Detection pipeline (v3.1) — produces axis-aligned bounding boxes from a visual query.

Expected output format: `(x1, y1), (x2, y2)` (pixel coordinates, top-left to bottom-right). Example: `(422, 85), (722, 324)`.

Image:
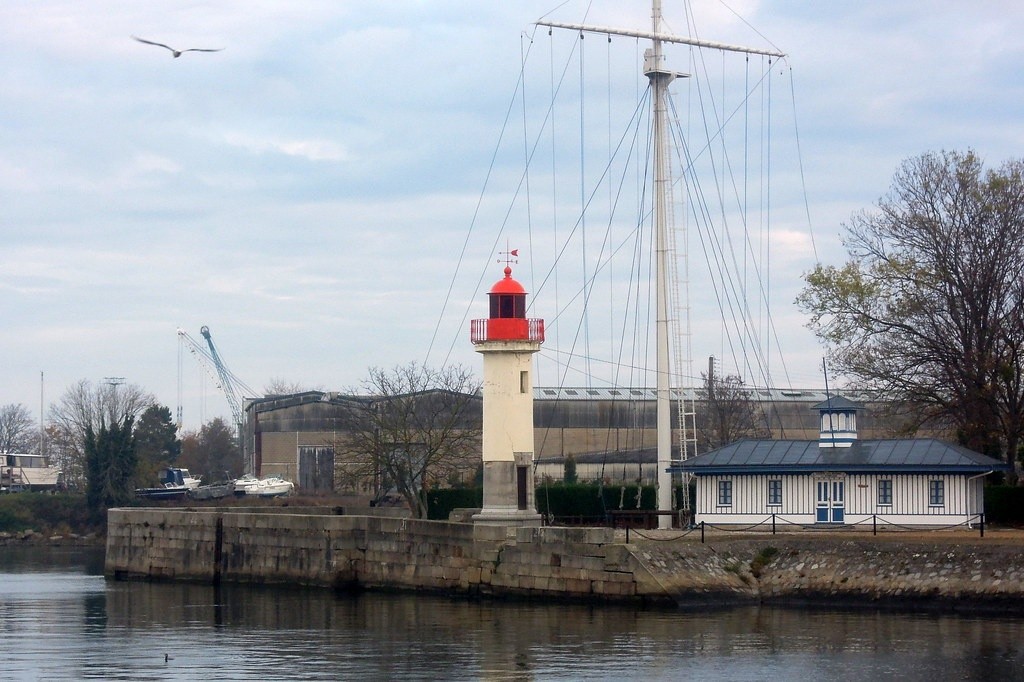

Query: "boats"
(0, 448), (296, 502)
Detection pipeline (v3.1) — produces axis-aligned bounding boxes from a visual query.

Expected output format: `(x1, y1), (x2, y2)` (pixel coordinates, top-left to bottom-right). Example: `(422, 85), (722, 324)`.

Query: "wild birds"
(164, 653), (175, 662)
(135, 36), (220, 58)
